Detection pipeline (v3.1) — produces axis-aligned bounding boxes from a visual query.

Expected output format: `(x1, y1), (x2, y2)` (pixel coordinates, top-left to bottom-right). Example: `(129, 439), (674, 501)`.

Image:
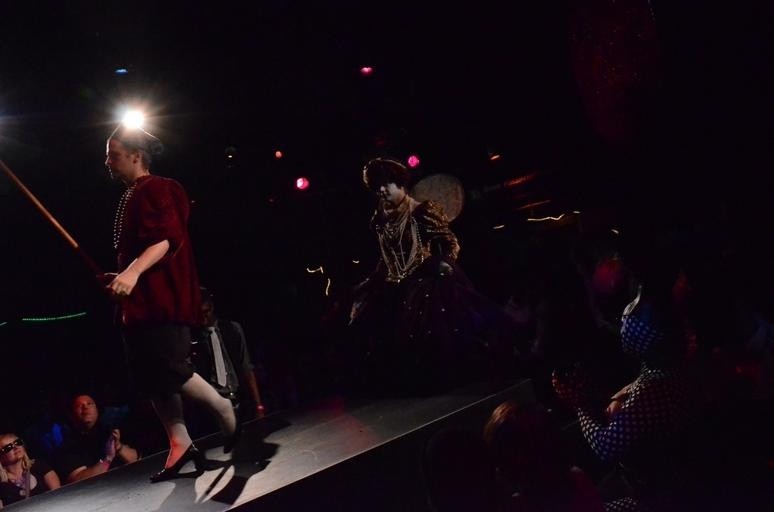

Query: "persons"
(346, 156), (462, 399)
(0, 256), (773, 512)
(95, 118), (239, 483)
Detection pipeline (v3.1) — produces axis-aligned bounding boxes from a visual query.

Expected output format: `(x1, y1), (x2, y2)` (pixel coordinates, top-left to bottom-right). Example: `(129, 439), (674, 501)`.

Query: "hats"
(360, 159), (410, 188)
(109, 123), (167, 159)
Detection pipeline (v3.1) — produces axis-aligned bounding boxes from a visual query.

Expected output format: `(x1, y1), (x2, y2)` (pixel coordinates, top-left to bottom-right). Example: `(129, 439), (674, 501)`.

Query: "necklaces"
(112, 184), (136, 250)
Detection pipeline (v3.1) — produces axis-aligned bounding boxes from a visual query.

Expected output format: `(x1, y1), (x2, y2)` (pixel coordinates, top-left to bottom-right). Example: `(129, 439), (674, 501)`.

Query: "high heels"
(224, 395), (243, 454)
(149, 442), (202, 482)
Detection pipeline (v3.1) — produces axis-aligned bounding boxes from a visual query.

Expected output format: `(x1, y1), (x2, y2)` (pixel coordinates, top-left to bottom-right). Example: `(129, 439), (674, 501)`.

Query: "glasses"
(0, 439), (24, 455)
